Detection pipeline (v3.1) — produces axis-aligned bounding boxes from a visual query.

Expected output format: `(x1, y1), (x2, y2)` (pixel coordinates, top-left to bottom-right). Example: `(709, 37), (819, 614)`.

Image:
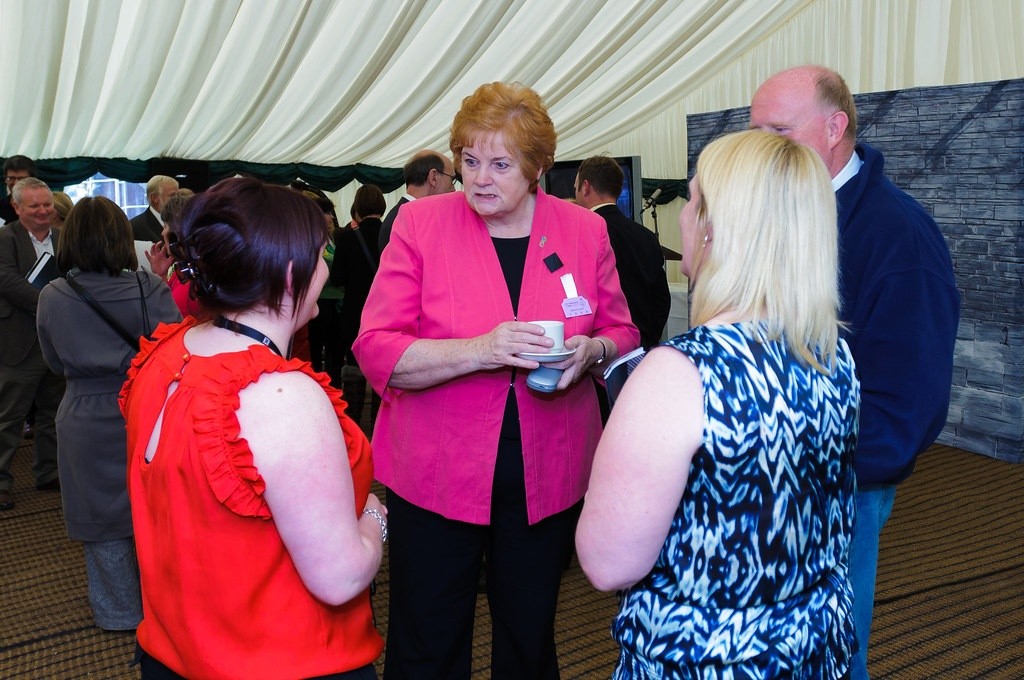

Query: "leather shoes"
(37, 476), (61, 491)
(0, 490), (16, 511)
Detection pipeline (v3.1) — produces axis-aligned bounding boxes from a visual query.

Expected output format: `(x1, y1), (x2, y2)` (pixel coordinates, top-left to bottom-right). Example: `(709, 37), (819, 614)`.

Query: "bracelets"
(363, 507), (388, 543)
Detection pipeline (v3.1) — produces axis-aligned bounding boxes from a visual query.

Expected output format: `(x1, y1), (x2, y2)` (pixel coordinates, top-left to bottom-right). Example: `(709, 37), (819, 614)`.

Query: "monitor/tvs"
(539, 156), (644, 226)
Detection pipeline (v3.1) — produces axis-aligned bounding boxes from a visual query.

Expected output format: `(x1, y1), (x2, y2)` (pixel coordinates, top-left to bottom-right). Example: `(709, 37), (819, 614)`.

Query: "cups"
(527, 321), (564, 354)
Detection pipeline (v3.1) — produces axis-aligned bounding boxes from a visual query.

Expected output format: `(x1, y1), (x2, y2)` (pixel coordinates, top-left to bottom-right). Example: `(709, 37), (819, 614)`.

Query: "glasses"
(436, 168), (458, 186)
(4, 176), (25, 184)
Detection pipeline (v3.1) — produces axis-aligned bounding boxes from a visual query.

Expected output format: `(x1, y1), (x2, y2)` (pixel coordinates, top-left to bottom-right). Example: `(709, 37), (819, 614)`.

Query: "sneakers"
(23, 421), (34, 440)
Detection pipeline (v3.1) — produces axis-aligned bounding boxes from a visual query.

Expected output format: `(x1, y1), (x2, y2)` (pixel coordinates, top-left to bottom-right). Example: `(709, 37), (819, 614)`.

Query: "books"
(25, 252), (58, 289)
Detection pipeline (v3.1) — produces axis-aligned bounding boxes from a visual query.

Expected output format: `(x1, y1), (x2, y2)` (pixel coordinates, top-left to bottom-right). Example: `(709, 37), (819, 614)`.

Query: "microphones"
(639, 189), (662, 215)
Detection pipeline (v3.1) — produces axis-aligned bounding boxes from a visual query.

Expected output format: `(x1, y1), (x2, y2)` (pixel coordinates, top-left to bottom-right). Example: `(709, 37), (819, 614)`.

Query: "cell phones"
(526, 366), (563, 392)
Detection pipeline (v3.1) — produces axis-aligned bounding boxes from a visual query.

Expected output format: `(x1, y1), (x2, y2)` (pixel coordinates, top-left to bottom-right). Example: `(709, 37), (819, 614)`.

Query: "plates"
(515, 346), (577, 363)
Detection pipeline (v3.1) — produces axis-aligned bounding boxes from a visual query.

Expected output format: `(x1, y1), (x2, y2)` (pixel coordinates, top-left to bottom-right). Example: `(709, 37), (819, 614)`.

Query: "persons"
(351, 82), (641, 680)
(378, 148), (457, 256)
(117, 177), (388, 680)
(0, 177), (73, 510)
(145, 188), (201, 317)
(0, 155), (36, 227)
(573, 156), (672, 354)
(751, 65), (962, 680)
(574, 128), (861, 680)
(36, 196), (183, 631)
(129, 175), (179, 250)
(290, 180), (381, 439)
